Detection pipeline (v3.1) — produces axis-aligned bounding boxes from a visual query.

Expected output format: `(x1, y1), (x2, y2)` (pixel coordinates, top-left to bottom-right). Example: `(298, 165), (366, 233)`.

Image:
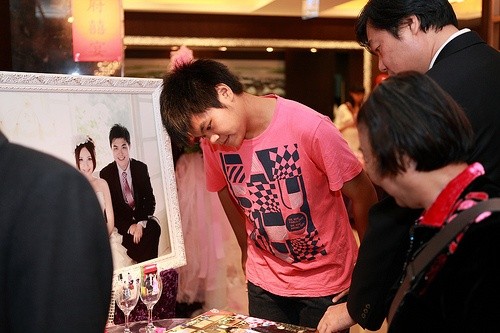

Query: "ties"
(122, 172), (134, 210)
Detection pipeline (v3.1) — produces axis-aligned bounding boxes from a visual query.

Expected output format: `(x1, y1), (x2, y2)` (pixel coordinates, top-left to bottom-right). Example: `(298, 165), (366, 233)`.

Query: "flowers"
(74, 134), (89, 148)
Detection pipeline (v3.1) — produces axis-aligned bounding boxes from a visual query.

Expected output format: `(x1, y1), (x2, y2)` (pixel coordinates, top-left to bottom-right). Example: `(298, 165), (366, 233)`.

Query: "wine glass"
(115, 277), (141, 333)
(139, 274), (164, 333)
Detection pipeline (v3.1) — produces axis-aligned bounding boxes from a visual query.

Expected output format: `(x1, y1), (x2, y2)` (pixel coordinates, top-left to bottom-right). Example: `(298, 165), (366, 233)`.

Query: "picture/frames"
(0, 71), (187, 278)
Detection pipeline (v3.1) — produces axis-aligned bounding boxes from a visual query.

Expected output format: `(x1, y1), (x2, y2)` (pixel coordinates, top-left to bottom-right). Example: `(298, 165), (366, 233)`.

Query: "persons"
(226, 325), (298, 333)
(74, 124), (161, 270)
(0, 132), (113, 333)
(316, 0), (500, 333)
(159, 57), (378, 333)
